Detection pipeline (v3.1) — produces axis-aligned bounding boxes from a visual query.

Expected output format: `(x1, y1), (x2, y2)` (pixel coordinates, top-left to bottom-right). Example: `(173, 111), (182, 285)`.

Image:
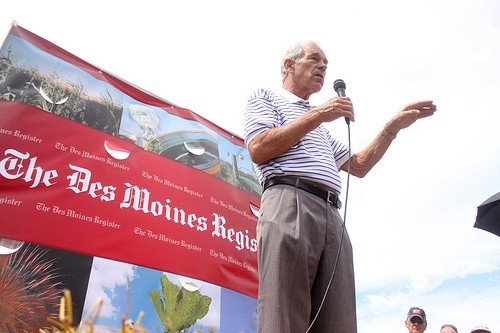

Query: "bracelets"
(382, 128), (397, 140)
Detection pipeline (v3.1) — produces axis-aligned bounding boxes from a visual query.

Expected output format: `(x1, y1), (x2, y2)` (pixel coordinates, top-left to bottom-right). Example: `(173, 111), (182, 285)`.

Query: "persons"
(440, 324), (458, 333)
(470, 325), (492, 333)
(243, 38), (438, 333)
(405, 306), (428, 333)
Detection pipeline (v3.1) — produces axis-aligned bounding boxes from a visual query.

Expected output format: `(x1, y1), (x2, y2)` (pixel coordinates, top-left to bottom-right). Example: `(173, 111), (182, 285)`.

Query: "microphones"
(333, 79), (350, 125)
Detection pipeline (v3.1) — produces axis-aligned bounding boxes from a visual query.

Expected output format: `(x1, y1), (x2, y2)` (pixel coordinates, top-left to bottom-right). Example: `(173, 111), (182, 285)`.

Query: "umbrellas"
(471, 192), (500, 237)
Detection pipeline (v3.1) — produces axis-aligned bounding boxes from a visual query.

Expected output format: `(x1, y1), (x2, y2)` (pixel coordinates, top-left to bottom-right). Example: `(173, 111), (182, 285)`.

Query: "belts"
(262, 178), (341, 208)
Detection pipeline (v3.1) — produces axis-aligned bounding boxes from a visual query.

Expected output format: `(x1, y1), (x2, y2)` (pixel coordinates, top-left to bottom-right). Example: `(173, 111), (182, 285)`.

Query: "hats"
(470, 326), (491, 333)
(407, 306), (426, 322)
